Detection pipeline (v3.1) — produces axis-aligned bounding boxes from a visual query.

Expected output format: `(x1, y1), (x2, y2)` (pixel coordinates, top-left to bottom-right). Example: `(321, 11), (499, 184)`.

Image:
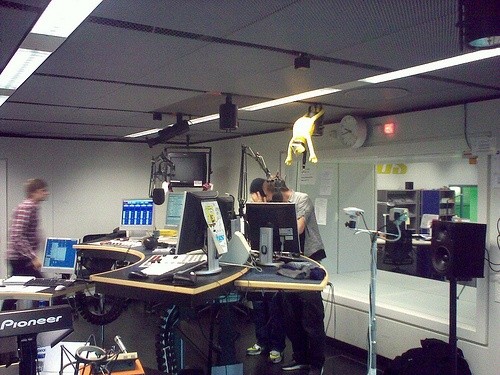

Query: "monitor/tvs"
(41, 238), (81, 279)
(245, 201), (301, 266)
(163, 191), (234, 275)
(120, 199), (154, 232)
(167, 151), (207, 186)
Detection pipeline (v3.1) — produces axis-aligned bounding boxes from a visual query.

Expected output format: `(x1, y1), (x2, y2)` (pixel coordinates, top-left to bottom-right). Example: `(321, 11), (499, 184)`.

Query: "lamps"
(455, 0), (500, 54)
(143, 118), (191, 148)
(219, 94), (239, 133)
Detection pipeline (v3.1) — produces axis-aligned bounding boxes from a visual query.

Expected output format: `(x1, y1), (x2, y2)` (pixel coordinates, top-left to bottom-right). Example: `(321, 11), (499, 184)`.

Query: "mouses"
(128, 271), (149, 280)
(54, 285), (66, 291)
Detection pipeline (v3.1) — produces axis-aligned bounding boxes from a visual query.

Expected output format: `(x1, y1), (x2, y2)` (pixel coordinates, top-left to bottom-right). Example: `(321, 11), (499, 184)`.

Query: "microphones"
(153, 173), (165, 205)
(272, 179), (283, 202)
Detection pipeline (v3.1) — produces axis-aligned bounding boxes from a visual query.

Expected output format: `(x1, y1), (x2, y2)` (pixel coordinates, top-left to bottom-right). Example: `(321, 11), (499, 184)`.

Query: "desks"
(377, 236), (431, 245)
(0, 232), (328, 375)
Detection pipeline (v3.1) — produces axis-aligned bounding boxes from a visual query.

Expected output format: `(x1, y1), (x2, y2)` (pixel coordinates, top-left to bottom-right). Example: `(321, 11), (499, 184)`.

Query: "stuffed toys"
(285, 108), (327, 167)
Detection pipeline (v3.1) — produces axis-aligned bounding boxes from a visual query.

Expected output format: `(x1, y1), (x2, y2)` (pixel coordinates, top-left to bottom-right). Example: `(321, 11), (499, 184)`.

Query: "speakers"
(430, 220), (487, 280)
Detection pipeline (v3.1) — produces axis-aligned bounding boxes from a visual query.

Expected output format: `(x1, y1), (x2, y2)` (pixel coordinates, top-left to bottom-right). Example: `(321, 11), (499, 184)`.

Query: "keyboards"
(153, 260), (207, 282)
(272, 254), (308, 264)
(23, 278), (76, 287)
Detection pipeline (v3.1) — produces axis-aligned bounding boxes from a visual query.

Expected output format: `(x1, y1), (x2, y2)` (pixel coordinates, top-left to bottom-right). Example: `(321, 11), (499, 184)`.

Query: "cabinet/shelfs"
(377, 189), (419, 233)
(418, 189), (456, 231)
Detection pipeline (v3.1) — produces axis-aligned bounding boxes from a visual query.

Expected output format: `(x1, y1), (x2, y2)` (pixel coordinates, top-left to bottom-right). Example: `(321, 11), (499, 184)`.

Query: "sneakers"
(268, 350), (284, 363)
(309, 365), (324, 375)
(247, 343), (269, 355)
(282, 357), (310, 370)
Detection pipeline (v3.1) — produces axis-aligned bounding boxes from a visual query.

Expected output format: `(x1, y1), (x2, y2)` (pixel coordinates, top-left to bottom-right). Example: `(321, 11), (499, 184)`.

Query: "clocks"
(340, 113), (367, 149)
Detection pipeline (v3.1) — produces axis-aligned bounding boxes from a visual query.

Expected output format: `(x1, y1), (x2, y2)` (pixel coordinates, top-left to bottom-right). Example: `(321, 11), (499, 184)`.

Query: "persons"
(1, 178), (49, 312)
(245, 178), (286, 362)
(262, 172), (327, 375)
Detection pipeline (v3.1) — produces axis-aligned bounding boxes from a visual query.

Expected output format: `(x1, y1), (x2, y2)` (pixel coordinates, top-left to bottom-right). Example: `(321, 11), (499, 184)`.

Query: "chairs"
(382, 231), (414, 274)
(75, 233), (122, 313)
